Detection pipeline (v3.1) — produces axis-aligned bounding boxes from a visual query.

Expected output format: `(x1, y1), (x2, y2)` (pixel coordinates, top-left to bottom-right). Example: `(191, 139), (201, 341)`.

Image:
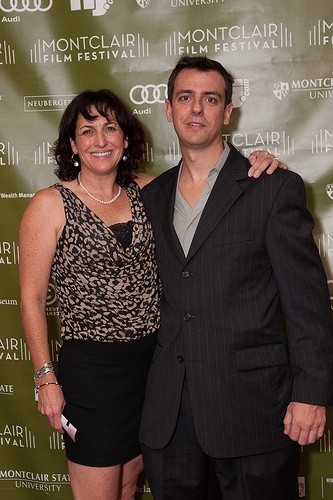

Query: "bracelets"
(36, 382), (62, 394)
(33, 360), (54, 381)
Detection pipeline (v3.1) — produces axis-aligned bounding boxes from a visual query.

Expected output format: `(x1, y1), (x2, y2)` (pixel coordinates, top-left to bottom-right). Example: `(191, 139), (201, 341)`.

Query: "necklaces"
(77, 170), (122, 205)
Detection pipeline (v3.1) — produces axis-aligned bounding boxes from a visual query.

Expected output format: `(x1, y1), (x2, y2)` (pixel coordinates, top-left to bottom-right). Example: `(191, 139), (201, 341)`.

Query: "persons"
(17, 88), (290, 500)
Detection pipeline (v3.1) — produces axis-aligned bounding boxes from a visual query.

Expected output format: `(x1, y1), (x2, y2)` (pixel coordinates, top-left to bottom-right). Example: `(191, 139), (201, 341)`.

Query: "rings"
(273, 157), (280, 162)
